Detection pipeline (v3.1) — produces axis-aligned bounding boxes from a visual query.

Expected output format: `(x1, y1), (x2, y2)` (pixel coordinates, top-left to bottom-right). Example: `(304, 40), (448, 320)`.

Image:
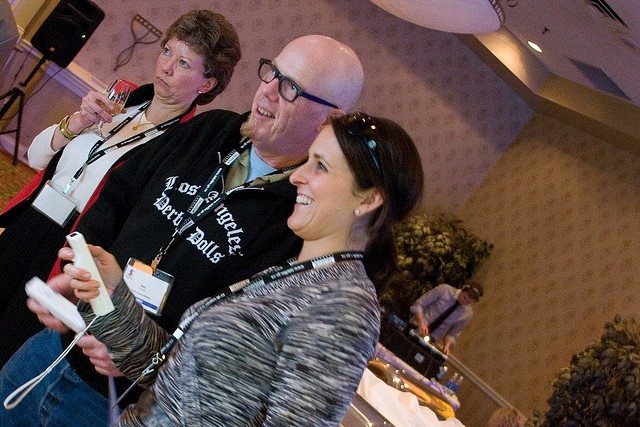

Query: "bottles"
(443, 372), (458, 388)
(454, 376), (464, 391)
(436, 366), (448, 379)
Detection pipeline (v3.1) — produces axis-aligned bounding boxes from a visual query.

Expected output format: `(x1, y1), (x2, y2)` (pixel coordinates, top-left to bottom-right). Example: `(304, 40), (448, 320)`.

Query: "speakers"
(31, 1), (106, 69)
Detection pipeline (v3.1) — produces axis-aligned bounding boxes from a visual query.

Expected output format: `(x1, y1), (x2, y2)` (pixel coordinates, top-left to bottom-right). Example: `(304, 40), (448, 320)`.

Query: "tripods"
(2, 54), (52, 169)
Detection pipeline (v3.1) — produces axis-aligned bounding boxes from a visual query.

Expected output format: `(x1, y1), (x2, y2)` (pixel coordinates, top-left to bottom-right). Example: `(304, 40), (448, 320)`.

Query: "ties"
(428, 299), (461, 334)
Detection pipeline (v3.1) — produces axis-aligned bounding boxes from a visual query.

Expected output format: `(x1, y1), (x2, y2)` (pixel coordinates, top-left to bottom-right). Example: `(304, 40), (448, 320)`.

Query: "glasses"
(257, 56), (340, 112)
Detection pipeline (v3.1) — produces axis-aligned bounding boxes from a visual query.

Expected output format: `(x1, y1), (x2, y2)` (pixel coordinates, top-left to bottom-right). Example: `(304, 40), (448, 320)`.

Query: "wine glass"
(88, 78), (132, 140)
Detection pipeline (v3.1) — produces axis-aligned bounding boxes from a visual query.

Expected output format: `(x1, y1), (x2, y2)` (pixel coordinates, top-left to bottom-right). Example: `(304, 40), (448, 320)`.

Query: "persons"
(410, 280), (484, 357)
(57, 112), (425, 427)
(0, 34), (364, 427)
(1, 10), (242, 365)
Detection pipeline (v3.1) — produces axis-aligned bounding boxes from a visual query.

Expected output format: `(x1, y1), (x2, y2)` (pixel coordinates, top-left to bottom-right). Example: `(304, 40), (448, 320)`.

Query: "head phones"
(462, 284), (481, 297)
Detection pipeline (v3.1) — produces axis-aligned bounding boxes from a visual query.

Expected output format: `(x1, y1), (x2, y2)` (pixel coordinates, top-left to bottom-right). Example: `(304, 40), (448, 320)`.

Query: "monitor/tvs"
(389, 315), (407, 332)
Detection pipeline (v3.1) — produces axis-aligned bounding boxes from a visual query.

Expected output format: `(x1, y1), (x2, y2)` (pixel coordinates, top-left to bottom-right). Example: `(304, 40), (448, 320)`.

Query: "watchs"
(59, 115), (77, 141)
